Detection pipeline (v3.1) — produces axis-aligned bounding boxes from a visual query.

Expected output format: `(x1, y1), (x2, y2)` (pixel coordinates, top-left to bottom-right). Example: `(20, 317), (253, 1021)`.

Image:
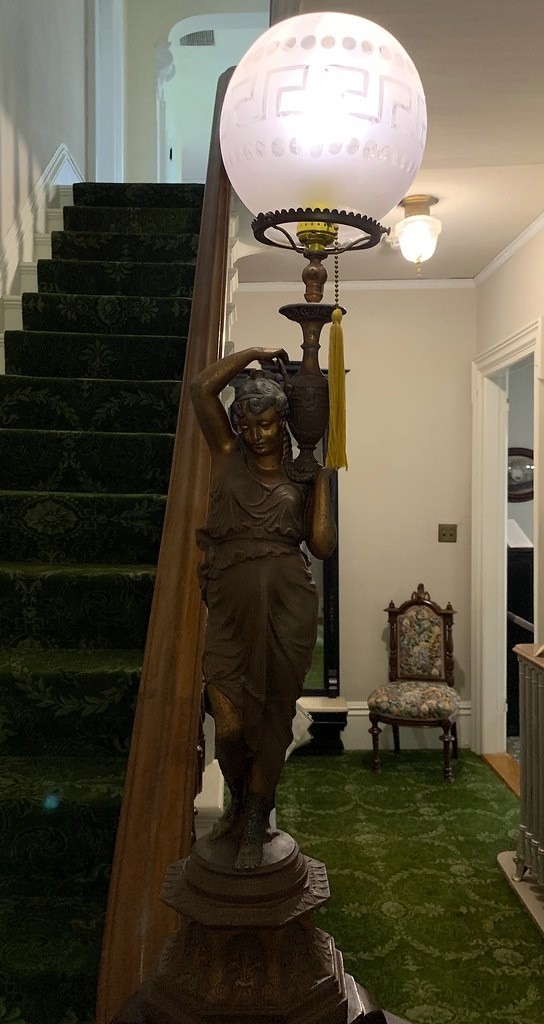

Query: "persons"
(189, 346), (337, 873)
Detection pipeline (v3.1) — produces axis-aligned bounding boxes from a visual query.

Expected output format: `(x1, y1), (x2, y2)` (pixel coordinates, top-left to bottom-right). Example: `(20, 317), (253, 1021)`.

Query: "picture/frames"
(506, 446), (534, 503)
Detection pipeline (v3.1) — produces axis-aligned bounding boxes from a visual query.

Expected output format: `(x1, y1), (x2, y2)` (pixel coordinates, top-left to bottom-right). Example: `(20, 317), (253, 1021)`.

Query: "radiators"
(513, 659), (544, 895)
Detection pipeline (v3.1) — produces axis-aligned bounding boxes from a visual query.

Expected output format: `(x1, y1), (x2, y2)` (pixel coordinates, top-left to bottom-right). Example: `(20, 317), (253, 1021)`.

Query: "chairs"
(368, 583), (458, 781)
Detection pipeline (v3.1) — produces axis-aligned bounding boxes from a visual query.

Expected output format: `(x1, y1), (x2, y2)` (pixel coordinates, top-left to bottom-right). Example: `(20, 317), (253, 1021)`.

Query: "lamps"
(220, 9), (429, 352)
(390, 195), (442, 277)
